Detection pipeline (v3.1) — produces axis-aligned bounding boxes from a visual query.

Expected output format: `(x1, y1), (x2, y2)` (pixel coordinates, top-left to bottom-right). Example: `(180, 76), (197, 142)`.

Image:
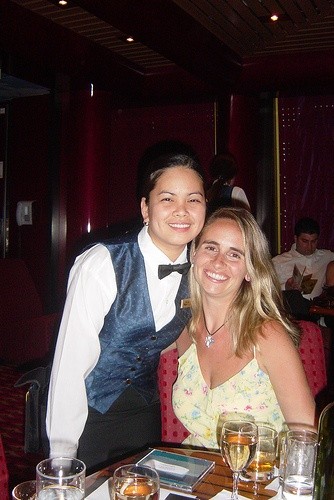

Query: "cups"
(111, 464), (161, 500)
(237, 424), (279, 481)
(274, 429), (318, 500)
(11, 456), (86, 500)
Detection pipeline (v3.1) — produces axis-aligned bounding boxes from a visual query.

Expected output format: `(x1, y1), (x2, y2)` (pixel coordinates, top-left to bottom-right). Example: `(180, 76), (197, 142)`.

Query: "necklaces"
(202, 303), (233, 349)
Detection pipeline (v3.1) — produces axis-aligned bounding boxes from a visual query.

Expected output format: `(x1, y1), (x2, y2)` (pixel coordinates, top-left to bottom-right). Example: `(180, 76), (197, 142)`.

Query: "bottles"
(313, 401), (334, 500)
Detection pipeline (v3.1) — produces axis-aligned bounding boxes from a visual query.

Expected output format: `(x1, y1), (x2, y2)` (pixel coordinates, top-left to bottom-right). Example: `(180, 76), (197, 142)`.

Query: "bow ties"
(158, 262), (192, 280)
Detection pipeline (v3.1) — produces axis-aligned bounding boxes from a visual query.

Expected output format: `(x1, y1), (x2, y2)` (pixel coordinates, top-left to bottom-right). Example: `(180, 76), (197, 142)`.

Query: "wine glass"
(220, 421), (258, 500)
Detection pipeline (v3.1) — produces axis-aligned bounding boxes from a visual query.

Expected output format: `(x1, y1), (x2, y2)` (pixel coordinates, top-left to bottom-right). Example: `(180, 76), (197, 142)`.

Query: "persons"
(171, 207), (319, 453)
(206, 152), (252, 213)
(44, 153), (207, 477)
(272, 217), (334, 327)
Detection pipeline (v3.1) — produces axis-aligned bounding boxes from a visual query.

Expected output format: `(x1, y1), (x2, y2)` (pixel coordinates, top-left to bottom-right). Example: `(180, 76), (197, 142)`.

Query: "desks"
(84, 446), (279, 500)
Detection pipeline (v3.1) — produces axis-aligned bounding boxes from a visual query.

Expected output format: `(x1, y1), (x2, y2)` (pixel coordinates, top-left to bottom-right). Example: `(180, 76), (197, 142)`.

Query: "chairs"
(0, 259), (330, 488)
